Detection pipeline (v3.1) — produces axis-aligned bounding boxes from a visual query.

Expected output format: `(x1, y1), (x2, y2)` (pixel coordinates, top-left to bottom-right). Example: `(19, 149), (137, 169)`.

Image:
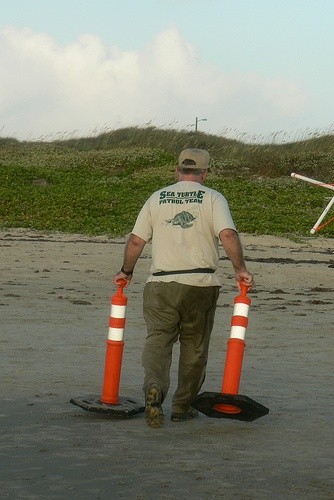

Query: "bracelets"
(121, 265), (133, 275)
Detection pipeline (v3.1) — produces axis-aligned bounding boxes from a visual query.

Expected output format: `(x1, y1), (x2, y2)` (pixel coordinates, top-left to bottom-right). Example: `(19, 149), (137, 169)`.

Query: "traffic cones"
(187, 281), (269, 422)
(69, 279), (146, 415)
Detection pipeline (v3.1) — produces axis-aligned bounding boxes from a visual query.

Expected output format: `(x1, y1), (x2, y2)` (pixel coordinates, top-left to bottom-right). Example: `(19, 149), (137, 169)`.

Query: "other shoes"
(170, 405), (200, 423)
(144, 385), (164, 428)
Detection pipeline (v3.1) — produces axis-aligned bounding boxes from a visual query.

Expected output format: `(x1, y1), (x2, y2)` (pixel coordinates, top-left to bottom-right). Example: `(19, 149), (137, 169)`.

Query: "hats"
(179, 148), (210, 170)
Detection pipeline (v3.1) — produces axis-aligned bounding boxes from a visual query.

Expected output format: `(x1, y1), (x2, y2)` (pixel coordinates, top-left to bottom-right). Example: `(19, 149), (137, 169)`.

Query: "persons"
(113, 148), (256, 428)
